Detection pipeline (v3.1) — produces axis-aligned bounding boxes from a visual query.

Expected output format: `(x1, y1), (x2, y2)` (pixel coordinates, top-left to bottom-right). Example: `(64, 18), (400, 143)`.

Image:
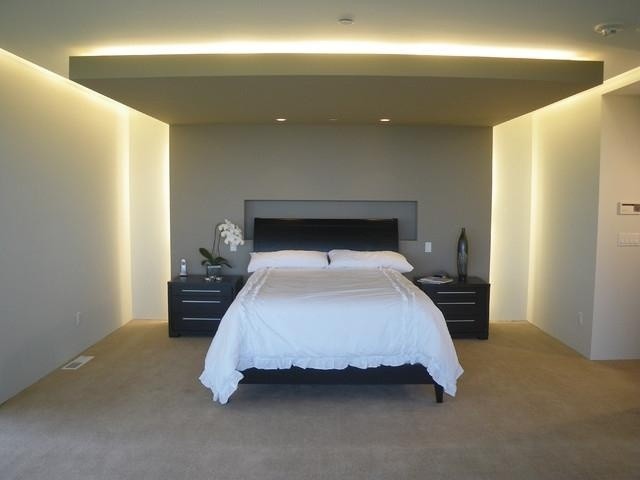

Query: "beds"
(198, 217), (467, 404)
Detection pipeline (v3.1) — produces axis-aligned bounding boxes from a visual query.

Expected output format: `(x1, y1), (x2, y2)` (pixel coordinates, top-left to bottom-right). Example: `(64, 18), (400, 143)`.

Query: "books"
(417, 276), (453, 285)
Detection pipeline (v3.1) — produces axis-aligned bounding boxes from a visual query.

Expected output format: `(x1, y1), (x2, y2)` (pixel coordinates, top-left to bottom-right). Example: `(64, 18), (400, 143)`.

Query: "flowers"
(197, 216), (245, 269)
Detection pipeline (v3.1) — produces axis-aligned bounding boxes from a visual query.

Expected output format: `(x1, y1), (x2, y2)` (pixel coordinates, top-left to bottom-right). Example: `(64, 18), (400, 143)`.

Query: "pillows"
(248, 248), (329, 273)
(326, 248), (414, 274)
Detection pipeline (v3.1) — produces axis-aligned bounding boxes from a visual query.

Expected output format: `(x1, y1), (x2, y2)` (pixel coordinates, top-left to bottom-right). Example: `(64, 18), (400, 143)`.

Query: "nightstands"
(413, 277), (490, 339)
(168, 272), (245, 338)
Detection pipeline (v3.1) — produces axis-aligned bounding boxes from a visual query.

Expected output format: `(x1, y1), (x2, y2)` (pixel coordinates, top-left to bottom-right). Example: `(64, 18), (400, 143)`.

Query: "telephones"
(179, 259), (188, 277)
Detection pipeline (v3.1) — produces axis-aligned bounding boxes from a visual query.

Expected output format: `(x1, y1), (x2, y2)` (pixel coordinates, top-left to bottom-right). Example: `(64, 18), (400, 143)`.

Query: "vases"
(457, 225), (469, 281)
(207, 264), (221, 278)
